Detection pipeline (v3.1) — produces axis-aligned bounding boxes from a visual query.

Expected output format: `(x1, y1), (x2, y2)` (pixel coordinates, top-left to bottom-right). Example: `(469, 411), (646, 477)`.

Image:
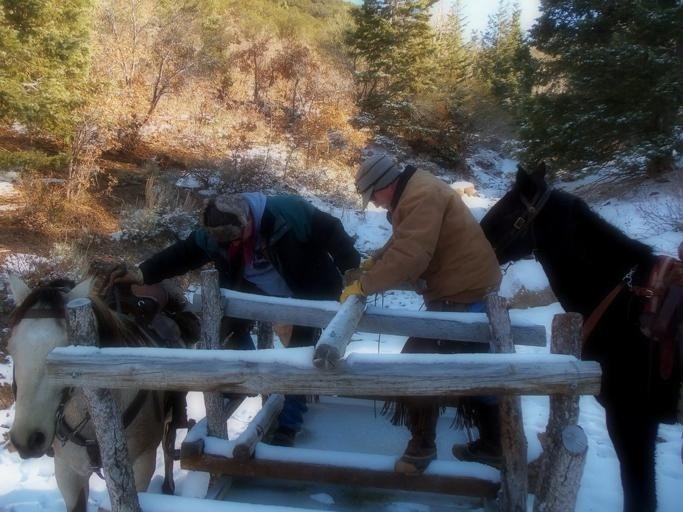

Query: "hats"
(204, 193), (249, 244)
(355, 152), (401, 210)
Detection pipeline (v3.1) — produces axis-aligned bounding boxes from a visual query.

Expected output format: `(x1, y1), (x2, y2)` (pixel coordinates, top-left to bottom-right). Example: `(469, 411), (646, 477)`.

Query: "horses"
(478, 160), (683, 512)
(5, 268), (193, 512)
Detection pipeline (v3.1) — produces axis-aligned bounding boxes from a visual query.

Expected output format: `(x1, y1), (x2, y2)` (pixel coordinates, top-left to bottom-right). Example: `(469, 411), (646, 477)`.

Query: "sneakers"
(394, 438), (436, 479)
(271, 423), (296, 448)
(452, 444), (505, 470)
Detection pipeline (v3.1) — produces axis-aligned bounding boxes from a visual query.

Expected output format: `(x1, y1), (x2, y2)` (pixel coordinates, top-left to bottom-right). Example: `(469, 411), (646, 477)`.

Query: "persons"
(340, 153), (504, 476)
(110, 192), (360, 444)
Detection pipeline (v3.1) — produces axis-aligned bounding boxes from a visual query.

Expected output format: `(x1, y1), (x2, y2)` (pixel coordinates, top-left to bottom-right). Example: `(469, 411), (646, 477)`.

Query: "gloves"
(339, 279), (367, 304)
